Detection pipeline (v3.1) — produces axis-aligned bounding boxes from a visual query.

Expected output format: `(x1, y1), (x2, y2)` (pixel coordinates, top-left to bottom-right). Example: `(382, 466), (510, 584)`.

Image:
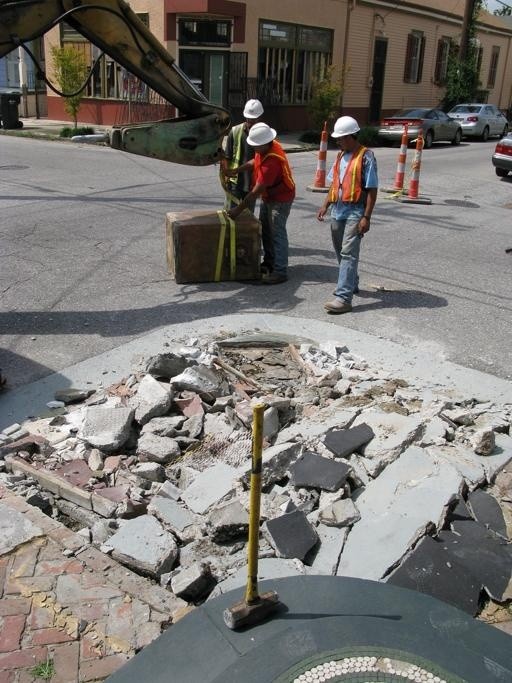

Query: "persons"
(316, 115), (378, 314)
(218, 99), (265, 213)
(225, 121), (296, 284)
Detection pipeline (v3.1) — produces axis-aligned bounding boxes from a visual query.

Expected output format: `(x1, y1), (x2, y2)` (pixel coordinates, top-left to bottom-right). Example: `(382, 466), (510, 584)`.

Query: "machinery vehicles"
(2, 0), (235, 167)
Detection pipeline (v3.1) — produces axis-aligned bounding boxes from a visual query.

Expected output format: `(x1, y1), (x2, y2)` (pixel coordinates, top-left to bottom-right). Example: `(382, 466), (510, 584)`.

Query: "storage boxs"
(163, 208), (262, 286)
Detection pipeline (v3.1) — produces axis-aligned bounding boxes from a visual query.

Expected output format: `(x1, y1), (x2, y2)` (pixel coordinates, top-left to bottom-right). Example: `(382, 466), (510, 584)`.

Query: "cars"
(446, 101), (509, 141)
(491, 131), (512, 177)
(376, 105), (461, 148)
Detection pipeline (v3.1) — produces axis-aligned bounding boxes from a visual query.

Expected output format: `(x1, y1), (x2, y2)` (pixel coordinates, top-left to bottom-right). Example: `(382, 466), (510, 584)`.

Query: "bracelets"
(362, 215), (371, 221)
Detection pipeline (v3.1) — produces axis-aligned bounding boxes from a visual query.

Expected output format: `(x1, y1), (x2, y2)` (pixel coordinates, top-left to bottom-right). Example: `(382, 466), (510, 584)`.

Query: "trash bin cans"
(507, 107), (512, 132)
(0, 92), (23, 130)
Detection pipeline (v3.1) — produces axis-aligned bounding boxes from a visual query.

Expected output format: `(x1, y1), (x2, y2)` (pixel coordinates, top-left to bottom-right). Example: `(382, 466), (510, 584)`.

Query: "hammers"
(222, 402), (280, 630)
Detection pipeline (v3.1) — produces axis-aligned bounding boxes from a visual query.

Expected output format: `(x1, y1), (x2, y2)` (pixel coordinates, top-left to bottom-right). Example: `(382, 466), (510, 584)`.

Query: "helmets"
(330, 115), (361, 138)
(243, 98), (265, 120)
(246, 122), (277, 146)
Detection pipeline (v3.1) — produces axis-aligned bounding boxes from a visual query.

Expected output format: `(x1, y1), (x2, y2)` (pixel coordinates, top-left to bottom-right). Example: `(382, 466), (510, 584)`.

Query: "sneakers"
(261, 262), (287, 284)
(324, 299), (352, 313)
(333, 284), (359, 296)
